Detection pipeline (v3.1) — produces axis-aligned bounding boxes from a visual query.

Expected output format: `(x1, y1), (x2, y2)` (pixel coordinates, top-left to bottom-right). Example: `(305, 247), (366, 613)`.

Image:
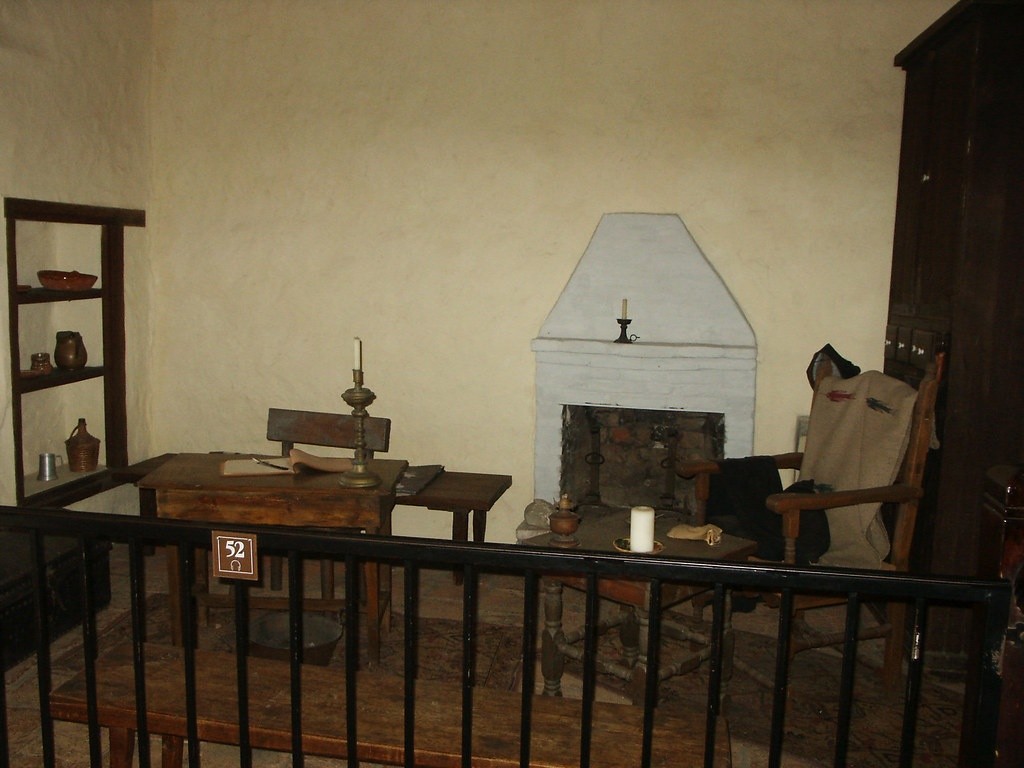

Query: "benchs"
(46, 641), (771, 768)
(111, 453), (513, 592)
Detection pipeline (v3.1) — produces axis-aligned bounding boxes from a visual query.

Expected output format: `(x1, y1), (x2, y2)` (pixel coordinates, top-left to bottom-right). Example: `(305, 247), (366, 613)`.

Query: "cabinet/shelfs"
(862, 0), (1024, 672)
(3, 196), (146, 510)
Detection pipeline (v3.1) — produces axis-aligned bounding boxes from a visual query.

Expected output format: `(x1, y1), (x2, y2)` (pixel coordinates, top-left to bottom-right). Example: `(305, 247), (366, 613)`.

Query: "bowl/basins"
(37, 270), (98, 291)
(249, 613), (344, 667)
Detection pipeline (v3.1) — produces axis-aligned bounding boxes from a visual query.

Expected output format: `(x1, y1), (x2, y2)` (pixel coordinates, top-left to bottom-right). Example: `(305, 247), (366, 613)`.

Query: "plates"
(613, 537), (664, 555)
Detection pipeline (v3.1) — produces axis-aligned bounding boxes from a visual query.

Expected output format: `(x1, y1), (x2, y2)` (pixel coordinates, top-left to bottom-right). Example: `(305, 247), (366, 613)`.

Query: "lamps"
(622, 297), (627, 320)
(354, 336), (361, 370)
(630, 505), (655, 553)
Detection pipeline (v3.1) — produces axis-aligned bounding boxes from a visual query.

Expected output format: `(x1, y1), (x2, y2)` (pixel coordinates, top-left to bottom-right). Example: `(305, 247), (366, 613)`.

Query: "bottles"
(65, 418), (101, 472)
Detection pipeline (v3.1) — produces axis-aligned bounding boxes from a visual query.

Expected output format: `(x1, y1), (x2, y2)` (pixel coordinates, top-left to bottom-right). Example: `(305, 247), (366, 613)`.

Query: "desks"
(135, 452), (409, 668)
(519, 509), (759, 715)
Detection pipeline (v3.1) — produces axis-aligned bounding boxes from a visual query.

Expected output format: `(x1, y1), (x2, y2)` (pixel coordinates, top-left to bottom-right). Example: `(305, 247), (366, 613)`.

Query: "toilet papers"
(629, 506), (654, 552)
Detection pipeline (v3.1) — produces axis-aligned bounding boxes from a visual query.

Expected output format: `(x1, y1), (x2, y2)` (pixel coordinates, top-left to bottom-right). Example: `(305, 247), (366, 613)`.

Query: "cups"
(37, 453), (63, 482)
(54, 331), (87, 371)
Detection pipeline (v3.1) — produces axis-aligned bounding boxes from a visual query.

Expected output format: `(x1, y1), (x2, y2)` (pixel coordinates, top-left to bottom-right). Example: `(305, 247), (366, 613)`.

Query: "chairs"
(267, 407), (393, 668)
(673, 352), (945, 733)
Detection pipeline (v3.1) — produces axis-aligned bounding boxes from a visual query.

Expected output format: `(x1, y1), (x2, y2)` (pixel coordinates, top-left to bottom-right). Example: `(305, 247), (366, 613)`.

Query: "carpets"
(532, 607), (962, 768)
(50, 589), (524, 697)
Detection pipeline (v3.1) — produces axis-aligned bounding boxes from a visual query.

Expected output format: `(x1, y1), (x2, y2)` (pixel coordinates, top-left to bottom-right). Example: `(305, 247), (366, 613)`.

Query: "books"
(220, 449), (354, 476)
